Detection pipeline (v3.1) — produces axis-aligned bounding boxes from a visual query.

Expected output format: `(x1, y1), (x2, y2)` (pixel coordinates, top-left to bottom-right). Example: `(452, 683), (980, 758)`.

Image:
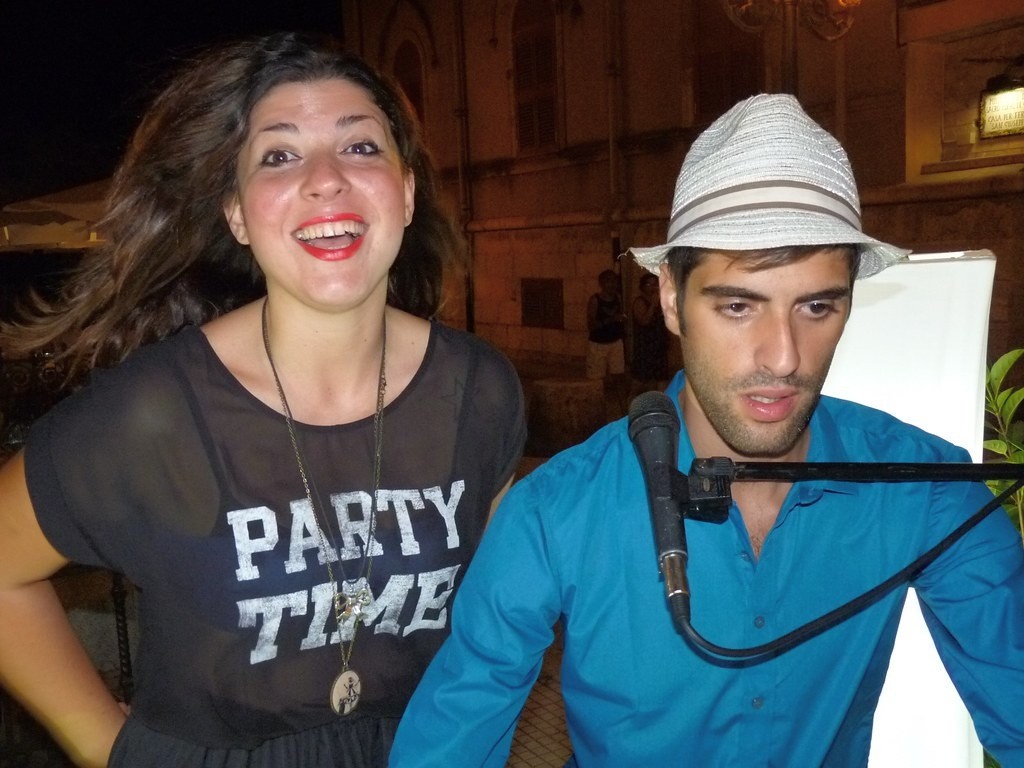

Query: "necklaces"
(262, 297), (389, 720)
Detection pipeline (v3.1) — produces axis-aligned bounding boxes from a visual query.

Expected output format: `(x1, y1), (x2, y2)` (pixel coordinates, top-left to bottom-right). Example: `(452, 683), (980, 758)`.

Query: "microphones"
(627, 392), (692, 623)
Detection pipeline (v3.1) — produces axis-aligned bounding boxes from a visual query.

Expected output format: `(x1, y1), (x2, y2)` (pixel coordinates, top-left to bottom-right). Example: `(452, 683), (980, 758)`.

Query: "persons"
(387, 95), (1024, 768)
(0, 34), (529, 768)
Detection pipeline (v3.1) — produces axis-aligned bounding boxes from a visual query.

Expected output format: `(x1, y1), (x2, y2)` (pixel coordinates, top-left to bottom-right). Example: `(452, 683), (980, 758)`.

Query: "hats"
(627, 93), (913, 283)
